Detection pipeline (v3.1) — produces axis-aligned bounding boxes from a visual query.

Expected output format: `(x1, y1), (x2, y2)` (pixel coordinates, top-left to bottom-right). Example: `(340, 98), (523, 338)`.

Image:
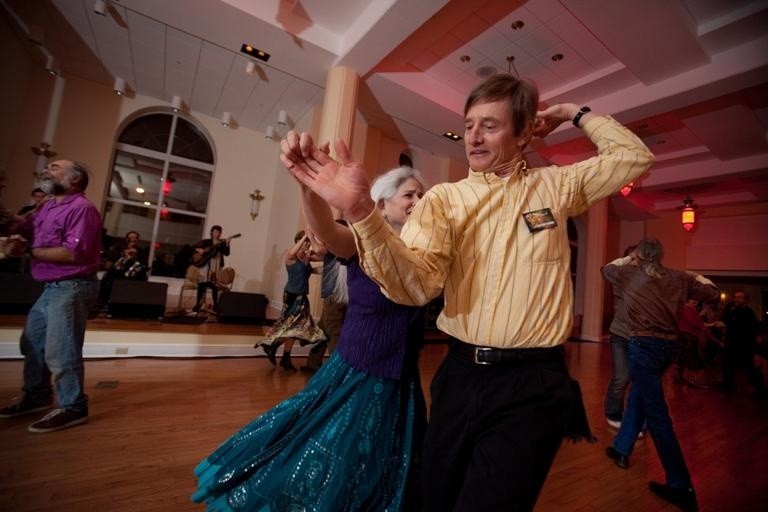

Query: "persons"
(189, 224), (232, 313)
(188, 129), (443, 511)
(597, 233), (768, 512)
(0, 188), (150, 321)
(300, 218), (352, 376)
(0, 158), (104, 433)
(278, 71), (658, 510)
(251, 225), (328, 375)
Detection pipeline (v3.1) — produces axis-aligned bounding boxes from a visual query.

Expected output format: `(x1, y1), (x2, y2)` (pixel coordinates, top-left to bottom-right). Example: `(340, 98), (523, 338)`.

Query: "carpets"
(0, 328), (331, 360)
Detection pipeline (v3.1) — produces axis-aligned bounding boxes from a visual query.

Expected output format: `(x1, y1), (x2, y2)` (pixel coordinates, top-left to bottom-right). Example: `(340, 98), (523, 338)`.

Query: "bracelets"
(571, 105), (592, 129)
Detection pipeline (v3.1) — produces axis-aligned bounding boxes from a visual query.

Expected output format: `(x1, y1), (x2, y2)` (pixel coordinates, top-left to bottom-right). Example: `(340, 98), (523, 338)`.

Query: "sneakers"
(27, 404), (88, 433)
(0, 389), (53, 418)
(300, 367), (316, 373)
(638, 431), (645, 439)
(605, 413), (623, 428)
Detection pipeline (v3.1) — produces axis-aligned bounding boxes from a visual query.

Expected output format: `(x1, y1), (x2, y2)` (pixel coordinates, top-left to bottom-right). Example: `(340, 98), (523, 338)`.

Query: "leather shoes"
(606, 446), (627, 468)
(649, 480), (697, 511)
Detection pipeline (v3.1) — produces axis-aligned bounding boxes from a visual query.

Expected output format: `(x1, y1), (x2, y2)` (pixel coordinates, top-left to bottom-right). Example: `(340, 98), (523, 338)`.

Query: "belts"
(448, 336), (558, 366)
(629, 330), (678, 341)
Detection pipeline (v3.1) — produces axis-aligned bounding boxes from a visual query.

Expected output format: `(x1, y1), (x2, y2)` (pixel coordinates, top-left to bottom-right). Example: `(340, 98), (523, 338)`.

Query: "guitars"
(192, 233), (242, 268)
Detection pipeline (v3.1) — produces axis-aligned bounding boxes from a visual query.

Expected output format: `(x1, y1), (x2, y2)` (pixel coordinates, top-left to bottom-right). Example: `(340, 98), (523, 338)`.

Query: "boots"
(265, 338), (282, 366)
(280, 351), (297, 372)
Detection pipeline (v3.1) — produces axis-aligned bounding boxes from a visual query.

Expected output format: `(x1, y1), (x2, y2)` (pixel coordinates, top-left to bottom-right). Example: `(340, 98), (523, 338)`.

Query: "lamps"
(620, 180), (634, 197)
(677, 194), (700, 233)
(31, 141), (57, 158)
(248, 189), (264, 221)
(28, 23), (45, 45)
(94, 0), (107, 16)
(245, 61), (255, 75)
(264, 126), (274, 145)
(171, 95), (182, 113)
(113, 77), (125, 96)
(277, 110), (288, 128)
(220, 111), (231, 128)
